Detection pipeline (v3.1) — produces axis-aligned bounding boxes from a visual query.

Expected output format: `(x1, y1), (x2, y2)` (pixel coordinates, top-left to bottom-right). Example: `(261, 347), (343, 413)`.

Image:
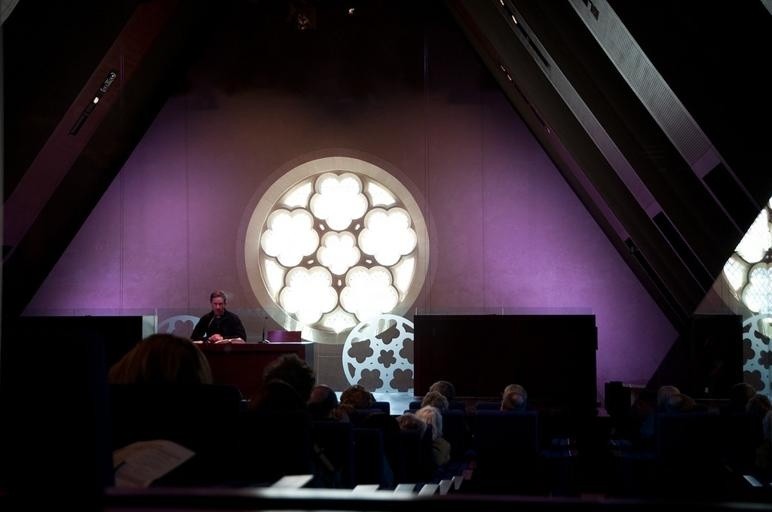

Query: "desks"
(0, 315), (158, 386)
(412, 313), (598, 413)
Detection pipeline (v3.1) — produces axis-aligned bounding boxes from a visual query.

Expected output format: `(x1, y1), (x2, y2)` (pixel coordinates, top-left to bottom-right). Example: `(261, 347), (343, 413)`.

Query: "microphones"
(203, 314), (218, 344)
(262, 317), (267, 341)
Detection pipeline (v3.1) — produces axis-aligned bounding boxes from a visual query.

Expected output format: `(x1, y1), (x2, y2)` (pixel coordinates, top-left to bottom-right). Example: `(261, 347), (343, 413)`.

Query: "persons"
(190, 291), (247, 343)
(1, 334), (771, 510)
